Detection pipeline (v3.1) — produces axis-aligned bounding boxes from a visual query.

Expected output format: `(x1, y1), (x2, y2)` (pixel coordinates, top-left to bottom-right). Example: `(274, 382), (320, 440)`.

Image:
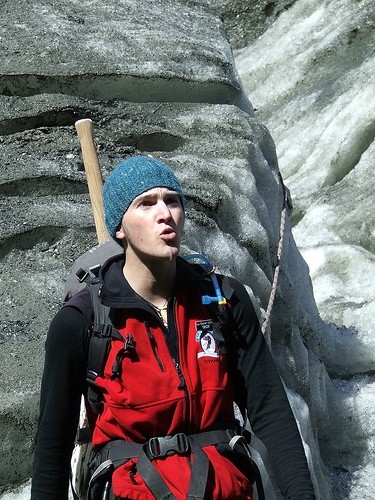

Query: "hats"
(103, 156), (186, 248)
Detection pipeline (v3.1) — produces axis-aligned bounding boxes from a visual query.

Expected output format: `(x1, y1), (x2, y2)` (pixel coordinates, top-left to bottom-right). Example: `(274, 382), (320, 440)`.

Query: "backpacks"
(64, 240), (266, 500)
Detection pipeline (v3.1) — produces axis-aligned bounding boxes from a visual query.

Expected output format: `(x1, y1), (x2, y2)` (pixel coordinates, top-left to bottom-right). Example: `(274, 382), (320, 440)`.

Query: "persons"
(31, 155), (317, 500)
